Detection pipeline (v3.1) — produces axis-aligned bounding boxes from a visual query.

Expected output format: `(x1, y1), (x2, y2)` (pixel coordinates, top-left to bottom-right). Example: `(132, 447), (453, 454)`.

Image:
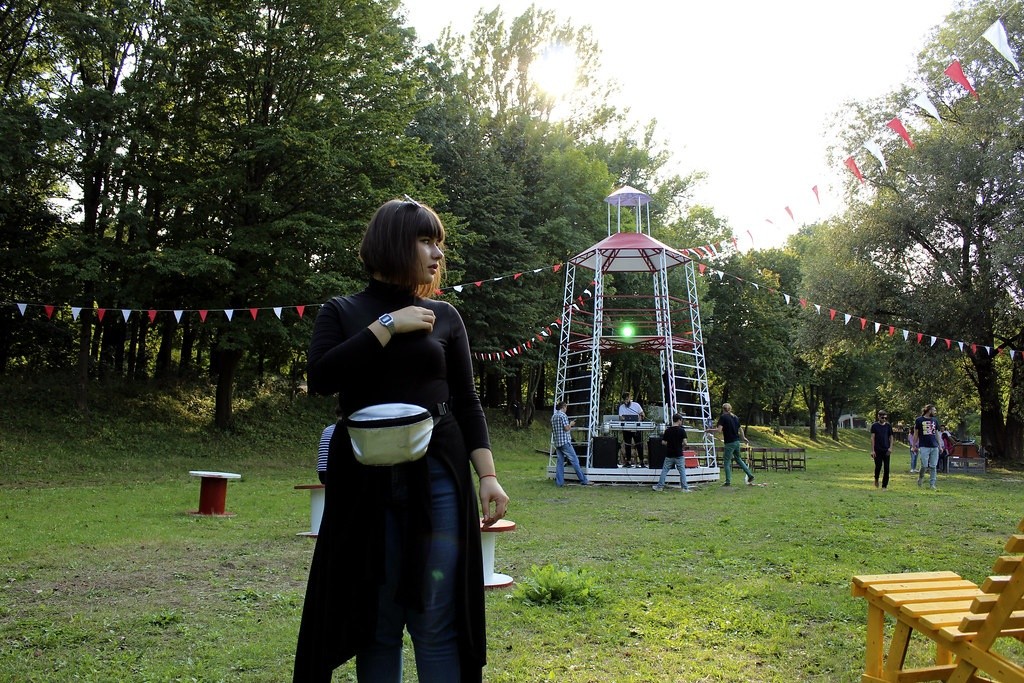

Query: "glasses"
(394, 194), (421, 214)
(879, 417), (888, 419)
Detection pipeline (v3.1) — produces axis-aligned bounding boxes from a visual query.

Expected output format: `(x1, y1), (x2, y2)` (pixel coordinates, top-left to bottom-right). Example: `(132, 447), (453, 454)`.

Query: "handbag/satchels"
(337, 400), (451, 467)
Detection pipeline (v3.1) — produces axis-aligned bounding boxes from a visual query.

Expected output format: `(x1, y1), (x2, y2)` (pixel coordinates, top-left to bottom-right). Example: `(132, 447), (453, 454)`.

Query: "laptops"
(622, 415), (638, 422)
(603, 415), (619, 421)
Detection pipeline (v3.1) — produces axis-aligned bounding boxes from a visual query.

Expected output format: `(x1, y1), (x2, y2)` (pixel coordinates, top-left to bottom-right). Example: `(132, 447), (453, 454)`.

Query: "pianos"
(606, 420), (656, 432)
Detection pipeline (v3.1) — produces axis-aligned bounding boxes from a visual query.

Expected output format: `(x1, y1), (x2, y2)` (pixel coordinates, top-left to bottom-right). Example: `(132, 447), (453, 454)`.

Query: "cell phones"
(573, 419), (577, 422)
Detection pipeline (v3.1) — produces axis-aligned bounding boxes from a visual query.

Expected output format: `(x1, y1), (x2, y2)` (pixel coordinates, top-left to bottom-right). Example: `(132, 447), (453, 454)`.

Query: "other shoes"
(931, 486), (937, 490)
(682, 487), (691, 493)
(883, 486), (888, 491)
(653, 486), (663, 492)
(749, 476), (756, 483)
(875, 478), (879, 487)
(917, 478), (923, 486)
(580, 481), (596, 486)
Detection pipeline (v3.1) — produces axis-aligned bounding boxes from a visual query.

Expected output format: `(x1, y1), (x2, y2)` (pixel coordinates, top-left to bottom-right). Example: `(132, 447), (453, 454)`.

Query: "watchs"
(378, 313), (396, 336)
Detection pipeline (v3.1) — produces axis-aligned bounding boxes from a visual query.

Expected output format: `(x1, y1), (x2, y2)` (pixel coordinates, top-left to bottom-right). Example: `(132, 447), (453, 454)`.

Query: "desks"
(477, 516), (516, 589)
(293, 484), (326, 536)
(187, 470), (242, 518)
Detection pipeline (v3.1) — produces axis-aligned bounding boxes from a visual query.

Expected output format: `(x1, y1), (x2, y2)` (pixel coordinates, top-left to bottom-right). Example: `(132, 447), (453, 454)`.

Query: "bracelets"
(479, 474), (496, 482)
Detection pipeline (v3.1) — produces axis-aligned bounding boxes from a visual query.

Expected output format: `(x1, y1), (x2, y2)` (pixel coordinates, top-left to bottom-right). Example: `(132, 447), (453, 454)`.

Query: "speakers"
(592, 437), (619, 468)
(648, 436), (676, 469)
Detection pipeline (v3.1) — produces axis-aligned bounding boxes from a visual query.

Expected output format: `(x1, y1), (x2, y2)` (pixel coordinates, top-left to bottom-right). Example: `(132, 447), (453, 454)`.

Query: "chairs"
(850, 517), (1024, 682)
(692, 444), (808, 471)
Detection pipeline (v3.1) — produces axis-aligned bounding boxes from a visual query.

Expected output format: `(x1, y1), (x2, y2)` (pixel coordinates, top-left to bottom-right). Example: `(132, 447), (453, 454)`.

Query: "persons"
(704, 403), (754, 486)
(292, 200), (510, 683)
(550, 402), (594, 487)
(652, 414), (693, 494)
(870, 410), (894, 488)
(618, 392), (648, 468)
(316, 405), (343, 484)
(908, 426), (920, 473)
(910, 404), (943, 490)
(938, 425), (956, 447)
(938, 433), (955, 472)
